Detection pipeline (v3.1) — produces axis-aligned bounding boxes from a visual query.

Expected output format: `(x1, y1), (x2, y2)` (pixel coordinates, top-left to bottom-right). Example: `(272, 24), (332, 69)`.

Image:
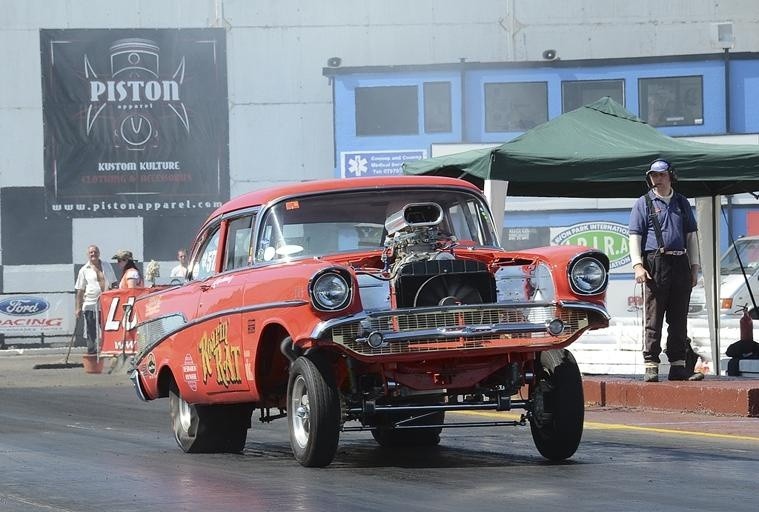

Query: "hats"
(111, 250), (138, 261)
(646, 161), (668, 175)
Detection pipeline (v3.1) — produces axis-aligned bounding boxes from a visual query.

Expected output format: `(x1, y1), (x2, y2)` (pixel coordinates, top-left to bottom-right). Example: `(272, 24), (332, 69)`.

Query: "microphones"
(650, 182), (662, 189)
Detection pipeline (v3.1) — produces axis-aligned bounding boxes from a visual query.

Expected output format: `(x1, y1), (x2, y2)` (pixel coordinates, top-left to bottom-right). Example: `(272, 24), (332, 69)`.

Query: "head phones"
(645, 158), (678, 189)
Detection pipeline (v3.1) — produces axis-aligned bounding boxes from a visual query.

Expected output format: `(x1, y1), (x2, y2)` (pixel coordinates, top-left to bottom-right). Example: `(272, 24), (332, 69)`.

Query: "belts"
(665, 250), (685, 255)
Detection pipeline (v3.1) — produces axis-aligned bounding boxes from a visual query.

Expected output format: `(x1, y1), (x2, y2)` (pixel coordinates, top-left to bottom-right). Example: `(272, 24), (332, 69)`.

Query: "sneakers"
(644, 366), (659, 382)
(668, 365), (704, 381)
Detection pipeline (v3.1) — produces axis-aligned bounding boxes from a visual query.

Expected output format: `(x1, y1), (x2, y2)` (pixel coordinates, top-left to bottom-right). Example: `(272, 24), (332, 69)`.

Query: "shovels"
(119, 303), (132, 371)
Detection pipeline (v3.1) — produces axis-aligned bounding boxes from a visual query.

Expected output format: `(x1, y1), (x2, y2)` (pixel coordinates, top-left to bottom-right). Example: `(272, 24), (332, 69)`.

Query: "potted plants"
(79, 333), (105, 374)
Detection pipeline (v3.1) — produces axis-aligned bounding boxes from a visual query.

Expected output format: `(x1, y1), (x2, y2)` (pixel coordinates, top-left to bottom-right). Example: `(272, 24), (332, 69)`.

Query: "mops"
(32, 307), (83, 370)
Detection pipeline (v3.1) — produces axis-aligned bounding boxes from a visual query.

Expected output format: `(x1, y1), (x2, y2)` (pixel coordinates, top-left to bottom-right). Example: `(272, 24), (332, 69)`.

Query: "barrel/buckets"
(82, 354), (104, 373)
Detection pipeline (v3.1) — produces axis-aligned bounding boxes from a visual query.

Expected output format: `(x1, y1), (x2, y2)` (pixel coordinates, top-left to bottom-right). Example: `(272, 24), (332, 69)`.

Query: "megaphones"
(542, 49), (557, 61)
(328, 56), (342, 67)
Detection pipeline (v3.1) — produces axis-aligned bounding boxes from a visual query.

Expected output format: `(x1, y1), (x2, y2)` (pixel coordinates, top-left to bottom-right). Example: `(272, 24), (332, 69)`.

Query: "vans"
(690, 233), (759, 317)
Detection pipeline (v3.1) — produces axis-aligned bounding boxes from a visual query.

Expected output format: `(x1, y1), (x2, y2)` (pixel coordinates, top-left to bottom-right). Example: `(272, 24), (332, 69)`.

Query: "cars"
(120, 166), (613, 468)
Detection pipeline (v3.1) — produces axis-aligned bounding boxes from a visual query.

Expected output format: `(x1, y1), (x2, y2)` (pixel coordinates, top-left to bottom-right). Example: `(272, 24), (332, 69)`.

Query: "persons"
(630, 161), (702, 381)
(170, 250), (203, 279)
(112, 249), (145, 288)
(75, 245), (116, 358)
